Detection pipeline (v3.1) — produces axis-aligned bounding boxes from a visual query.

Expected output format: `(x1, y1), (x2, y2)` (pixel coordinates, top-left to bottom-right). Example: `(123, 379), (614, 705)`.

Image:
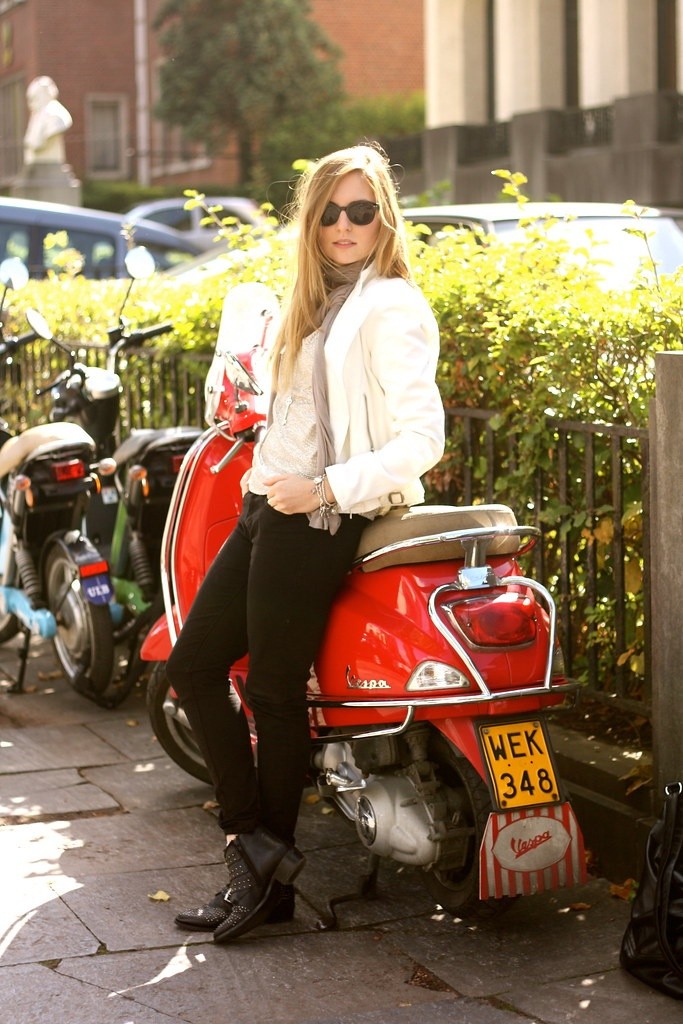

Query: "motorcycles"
(140, 278), (592, 932)
(23, 244), (210, 712)
(1, 257), (115, 697)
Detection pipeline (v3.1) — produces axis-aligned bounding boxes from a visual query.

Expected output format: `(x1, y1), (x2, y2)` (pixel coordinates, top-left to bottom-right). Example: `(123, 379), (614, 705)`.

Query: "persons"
(163, 138), (448, 946)
(19, 73), (75, 164)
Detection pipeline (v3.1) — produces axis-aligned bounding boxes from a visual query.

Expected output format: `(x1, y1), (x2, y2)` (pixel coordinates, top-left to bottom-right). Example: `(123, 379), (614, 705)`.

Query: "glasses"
(321, 199), (379, 226)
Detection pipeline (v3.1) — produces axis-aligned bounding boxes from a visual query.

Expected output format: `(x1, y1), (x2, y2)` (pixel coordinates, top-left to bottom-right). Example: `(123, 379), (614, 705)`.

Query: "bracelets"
(309, 474), (338, 520)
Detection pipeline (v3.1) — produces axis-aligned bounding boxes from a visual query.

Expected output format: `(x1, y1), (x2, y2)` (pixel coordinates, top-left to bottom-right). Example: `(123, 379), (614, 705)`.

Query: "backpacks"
(619, 780), (683, 1003)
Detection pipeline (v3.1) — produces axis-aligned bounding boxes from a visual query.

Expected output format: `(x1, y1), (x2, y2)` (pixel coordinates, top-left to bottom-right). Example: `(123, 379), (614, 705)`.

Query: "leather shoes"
(175, 875), (295, 932)
(213, 824), (306, 943)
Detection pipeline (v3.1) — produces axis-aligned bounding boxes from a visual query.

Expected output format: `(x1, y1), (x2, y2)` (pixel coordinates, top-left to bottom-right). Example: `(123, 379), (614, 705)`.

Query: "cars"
(125, 198), (271, 240)
(167, 199), (683, 287)
(1, 197), (214, 274)
